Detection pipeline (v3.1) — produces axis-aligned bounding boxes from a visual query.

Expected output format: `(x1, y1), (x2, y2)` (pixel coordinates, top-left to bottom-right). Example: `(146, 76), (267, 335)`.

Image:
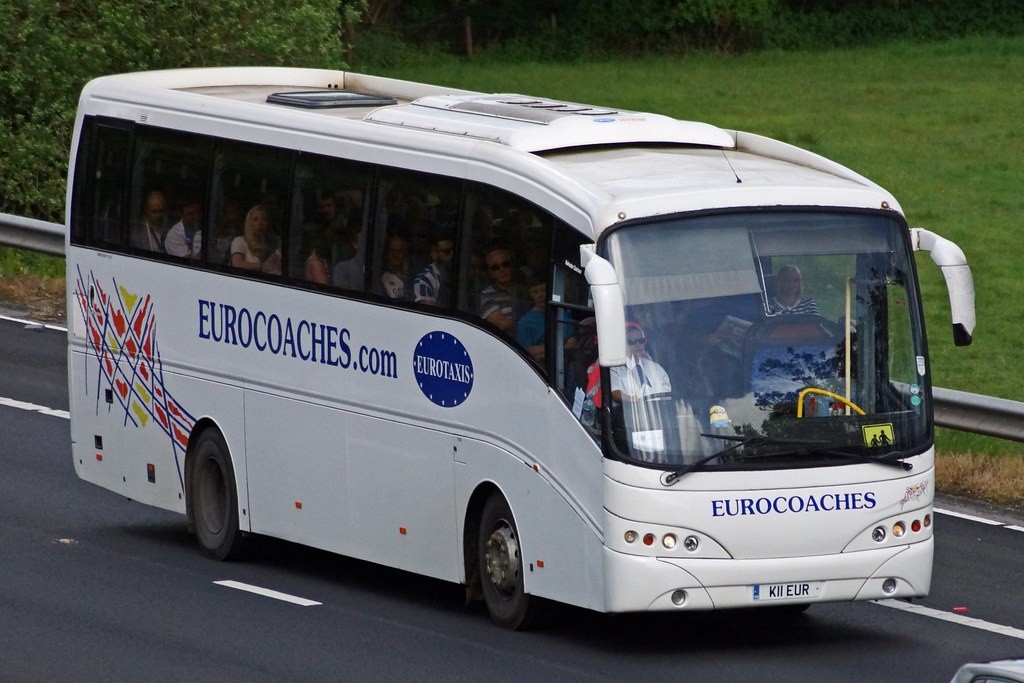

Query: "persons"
(515, 278), (578, 366)
(230, 204), (281, 275)
(479, 249), (515, 330)
(167, 193), (227, 265)
(609, 323), (672, 444)
(304, 193), (410, 303)
(413, 232), (455, 309)
(140, 191), (172, 252)
(762, 265), (820, 316)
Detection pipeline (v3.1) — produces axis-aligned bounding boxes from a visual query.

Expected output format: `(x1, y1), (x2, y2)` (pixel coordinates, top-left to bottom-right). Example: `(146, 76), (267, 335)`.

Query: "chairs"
(99, 170), (858, 455)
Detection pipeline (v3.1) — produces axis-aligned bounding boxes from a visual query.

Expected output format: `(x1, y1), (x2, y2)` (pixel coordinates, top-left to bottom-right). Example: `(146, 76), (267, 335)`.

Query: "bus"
(66, 64), (977, 630)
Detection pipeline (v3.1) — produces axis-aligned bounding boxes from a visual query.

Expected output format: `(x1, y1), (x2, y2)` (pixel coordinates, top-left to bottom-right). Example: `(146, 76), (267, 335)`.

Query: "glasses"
(491, 260), (512, 270)
(627, 339), (644, 345)
(434, 247), (456, 256)
(316, 203), (334, 210)
